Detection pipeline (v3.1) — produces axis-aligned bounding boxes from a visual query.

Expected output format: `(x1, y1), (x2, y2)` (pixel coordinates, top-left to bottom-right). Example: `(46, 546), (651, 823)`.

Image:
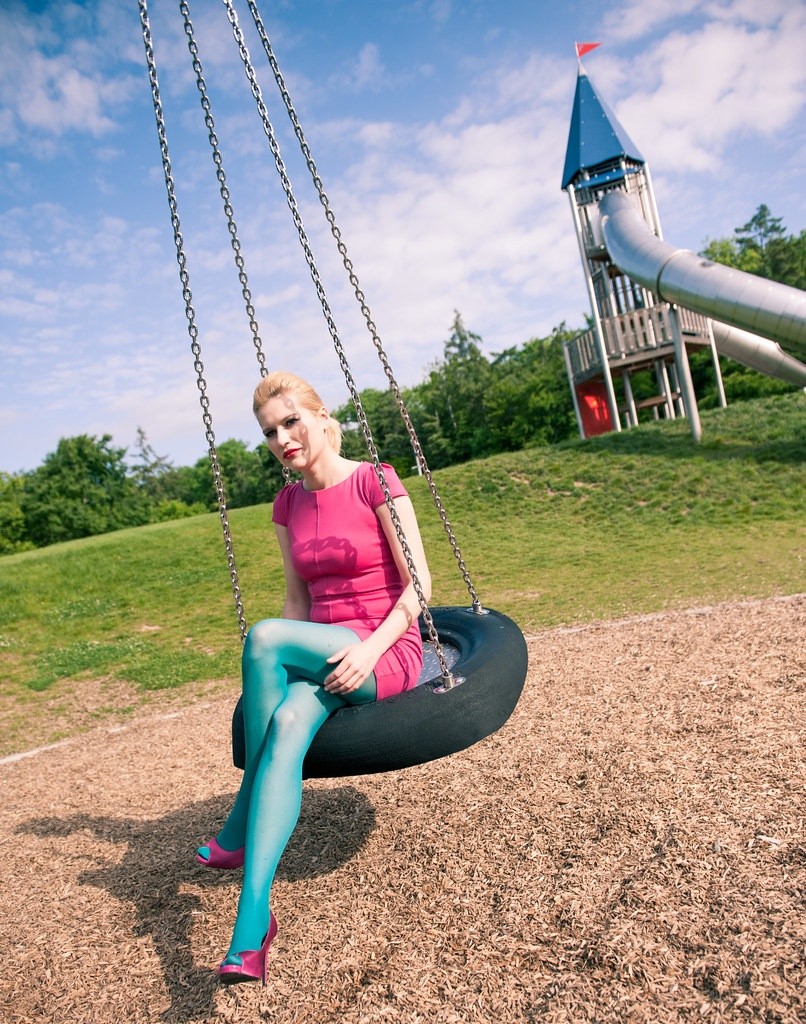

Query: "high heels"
(218, 910), (278, 985)
(197, 836), (244, 869)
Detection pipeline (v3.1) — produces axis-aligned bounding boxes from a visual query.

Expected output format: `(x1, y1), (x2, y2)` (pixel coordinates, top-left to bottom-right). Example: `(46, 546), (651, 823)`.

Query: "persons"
(195, 373), (431, 987)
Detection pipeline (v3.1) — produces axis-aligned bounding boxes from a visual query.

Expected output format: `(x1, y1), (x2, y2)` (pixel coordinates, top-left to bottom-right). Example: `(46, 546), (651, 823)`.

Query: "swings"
(134, 0), (530, 778)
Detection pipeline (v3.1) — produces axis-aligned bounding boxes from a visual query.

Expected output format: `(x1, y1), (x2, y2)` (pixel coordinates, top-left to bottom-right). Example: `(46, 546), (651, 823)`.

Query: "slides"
(599, 193), (805, 354)
(710, 319), (806, 389)
